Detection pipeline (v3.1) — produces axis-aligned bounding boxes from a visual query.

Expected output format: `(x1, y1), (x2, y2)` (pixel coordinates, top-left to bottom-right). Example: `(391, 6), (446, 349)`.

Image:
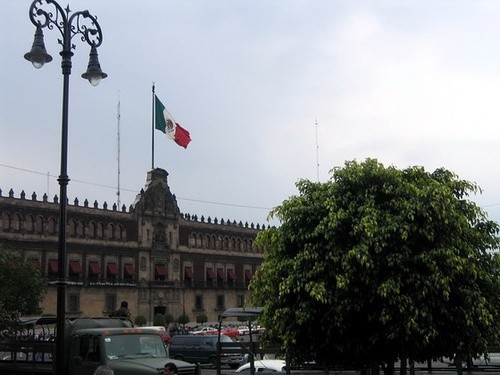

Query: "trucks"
(1, 315), (202, 375)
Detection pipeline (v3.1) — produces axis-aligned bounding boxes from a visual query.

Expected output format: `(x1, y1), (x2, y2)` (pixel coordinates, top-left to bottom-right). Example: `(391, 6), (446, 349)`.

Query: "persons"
(109, 300), (131, 319)
(168, 322), (263, 336)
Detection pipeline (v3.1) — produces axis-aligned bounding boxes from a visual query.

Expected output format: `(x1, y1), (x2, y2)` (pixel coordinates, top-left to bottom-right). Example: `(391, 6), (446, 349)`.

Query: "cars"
(235, 358), (287, 373)
(187, 324), (267, 338)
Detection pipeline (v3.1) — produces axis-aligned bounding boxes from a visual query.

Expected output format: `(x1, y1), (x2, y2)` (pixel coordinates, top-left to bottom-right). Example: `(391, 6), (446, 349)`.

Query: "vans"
(168, 334), (244, 370)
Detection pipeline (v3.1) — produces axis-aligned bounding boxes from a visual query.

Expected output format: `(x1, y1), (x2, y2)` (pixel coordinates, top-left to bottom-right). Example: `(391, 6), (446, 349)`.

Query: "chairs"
(121, 336), (141, 353)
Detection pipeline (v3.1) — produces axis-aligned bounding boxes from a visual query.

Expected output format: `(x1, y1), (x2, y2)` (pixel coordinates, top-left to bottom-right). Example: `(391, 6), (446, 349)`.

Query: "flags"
(154, 95), (192, 150)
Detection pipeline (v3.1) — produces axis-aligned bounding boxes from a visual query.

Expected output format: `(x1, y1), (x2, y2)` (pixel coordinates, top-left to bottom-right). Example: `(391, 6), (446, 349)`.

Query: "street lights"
(23, 0), (109, 375)
